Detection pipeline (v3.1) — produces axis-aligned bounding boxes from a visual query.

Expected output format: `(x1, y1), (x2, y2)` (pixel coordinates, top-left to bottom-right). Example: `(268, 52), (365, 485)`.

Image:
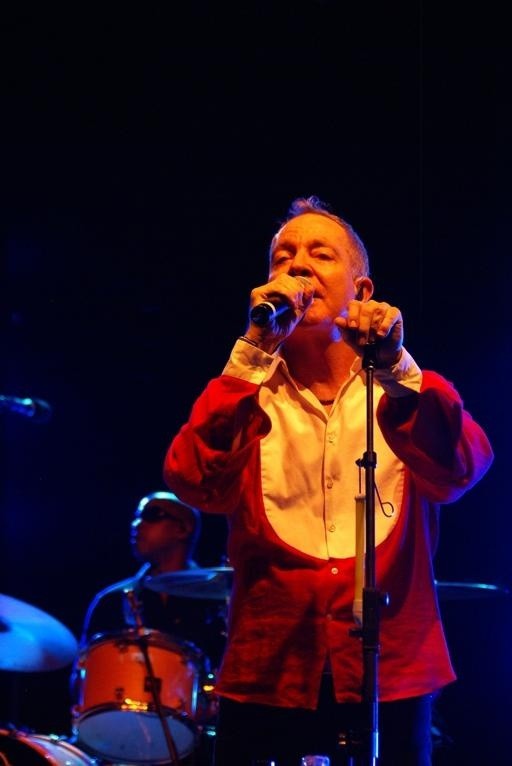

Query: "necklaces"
(317, 396), (337, 410)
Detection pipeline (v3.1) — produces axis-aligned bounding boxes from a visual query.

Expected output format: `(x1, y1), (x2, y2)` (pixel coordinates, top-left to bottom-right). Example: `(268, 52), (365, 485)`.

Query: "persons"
(162, 191), (492, 764)
(75, 491), (230, 683)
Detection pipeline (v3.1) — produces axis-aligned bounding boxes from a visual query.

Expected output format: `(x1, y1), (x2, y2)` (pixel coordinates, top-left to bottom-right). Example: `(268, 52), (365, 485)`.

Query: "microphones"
(251, 296), (292, 327)
(0, 394), (50, 424)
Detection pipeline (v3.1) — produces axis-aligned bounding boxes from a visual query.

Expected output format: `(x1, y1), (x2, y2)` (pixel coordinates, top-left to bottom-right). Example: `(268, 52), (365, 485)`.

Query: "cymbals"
(0, 593), (78, 673)
(143, 567), (231, 602)
(434, 581), (510, 594)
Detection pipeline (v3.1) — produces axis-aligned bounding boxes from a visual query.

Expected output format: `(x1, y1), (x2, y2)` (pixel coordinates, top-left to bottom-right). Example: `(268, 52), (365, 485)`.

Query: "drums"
(72, 628), (212, 763)
(0, 726), (96, 766)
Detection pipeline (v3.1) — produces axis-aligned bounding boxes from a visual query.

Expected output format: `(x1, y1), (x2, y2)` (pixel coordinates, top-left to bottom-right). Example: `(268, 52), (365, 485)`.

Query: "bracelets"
(238, 333), (260, 350)
